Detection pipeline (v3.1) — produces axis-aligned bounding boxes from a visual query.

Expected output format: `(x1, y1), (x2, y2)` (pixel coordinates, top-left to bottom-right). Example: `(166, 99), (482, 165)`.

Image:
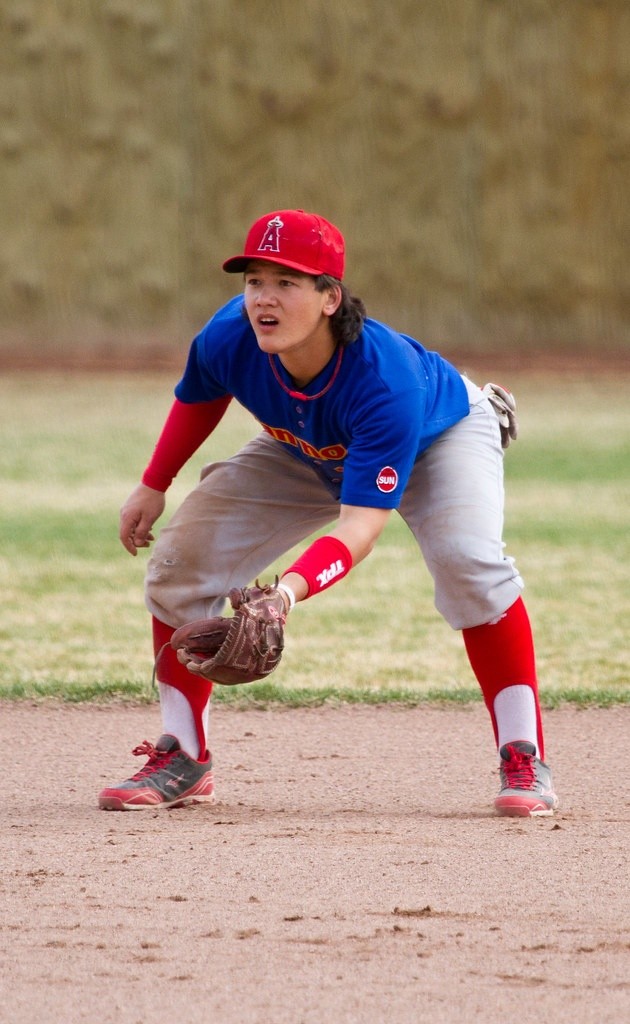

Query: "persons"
(98, 209), (559, 817)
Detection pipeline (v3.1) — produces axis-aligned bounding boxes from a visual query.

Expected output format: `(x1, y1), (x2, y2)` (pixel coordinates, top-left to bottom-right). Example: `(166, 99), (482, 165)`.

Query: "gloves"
(483, 382), (518, 447)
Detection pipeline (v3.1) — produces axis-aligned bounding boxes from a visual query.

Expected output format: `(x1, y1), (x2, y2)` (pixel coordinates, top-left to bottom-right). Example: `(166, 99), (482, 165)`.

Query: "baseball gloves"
(170, 579), (291, 686)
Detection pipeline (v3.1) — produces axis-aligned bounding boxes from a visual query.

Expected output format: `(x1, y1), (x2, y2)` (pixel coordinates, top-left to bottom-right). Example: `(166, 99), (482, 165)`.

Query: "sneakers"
(99, 734), (214, 811)
(495, 741), (559, 817)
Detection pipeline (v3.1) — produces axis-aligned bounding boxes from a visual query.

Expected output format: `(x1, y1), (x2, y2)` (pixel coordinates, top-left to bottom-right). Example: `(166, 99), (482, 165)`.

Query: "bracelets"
(272, 584), (295, 615)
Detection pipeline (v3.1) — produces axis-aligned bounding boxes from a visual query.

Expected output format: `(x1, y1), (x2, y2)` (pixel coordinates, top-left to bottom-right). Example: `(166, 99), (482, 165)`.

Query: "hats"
(222, 209), (344, 281)
